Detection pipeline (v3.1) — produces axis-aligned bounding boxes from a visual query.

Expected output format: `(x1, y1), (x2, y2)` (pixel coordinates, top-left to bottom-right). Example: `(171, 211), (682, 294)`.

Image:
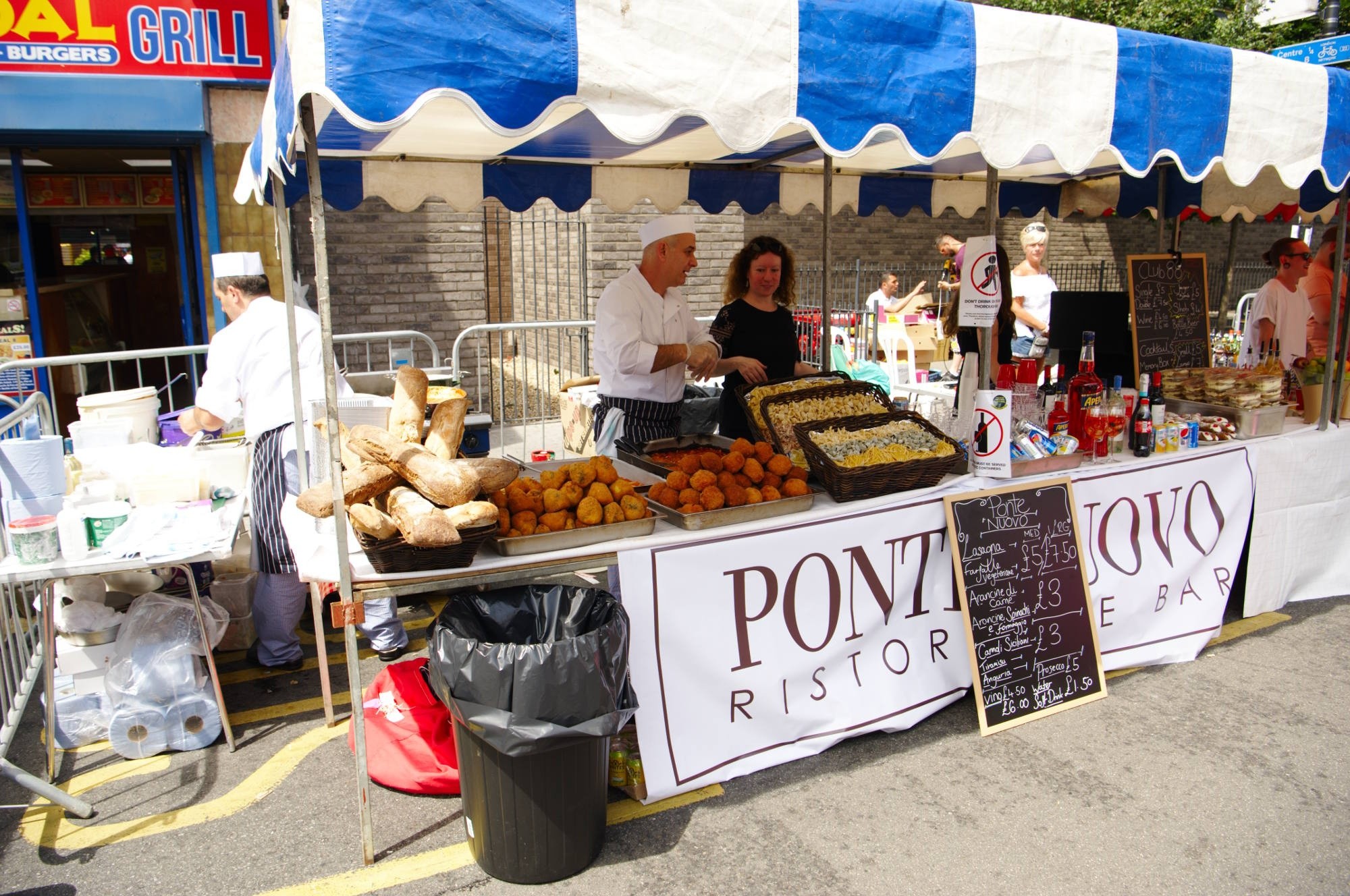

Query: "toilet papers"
(39, 639), (224, 763)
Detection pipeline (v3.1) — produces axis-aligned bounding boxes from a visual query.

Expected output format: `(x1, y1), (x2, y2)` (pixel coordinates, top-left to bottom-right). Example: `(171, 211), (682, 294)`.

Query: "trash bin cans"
(678, 385), (723, 433)
(434, 583), (625, 884)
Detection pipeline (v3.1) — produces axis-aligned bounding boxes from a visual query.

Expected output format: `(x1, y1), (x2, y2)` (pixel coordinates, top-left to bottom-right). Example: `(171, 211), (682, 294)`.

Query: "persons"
(934, 233), (966, 290)
(1010, 223), (1057, 362)
(709, 235), (818, 443)
(1299, 225), (1350, 361)
(863, 270), (927, 335)
(1238, 237), (1314, 381)
(595, 217), (722, 455)
(944, 245), (1016, 416)
(179, 253), (407, 670)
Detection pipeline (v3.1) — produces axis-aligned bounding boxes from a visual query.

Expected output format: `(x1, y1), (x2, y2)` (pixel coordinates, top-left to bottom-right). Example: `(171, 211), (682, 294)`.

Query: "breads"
(297, 366), (520, 546)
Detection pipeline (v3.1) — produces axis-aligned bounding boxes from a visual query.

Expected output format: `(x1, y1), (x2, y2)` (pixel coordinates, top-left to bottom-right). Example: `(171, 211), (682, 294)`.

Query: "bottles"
(1210, 327), (1286, 377)
(996, 331), (1166, 461)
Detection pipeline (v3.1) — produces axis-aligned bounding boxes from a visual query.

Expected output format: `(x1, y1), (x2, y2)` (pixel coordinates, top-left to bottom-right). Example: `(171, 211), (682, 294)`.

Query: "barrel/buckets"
(82, 501), (130, 549)
(78, 386), (161, 444)
(66, 419), (140, 472)
(9, 515), (59, 565)
(129, 444), (250, 547)
(156, 404), (220, 446)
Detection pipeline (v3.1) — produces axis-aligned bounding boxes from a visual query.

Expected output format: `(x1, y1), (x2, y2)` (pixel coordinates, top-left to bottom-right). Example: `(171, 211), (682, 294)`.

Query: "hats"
(1322, 225), (1350, 244)
(212, 251), (264, 278)
(639, 214), (698, 248)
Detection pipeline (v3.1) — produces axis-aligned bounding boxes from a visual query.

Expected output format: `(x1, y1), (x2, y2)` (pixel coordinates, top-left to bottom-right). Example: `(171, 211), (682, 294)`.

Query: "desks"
(289, 355), (1350, 869)
(895, 380), (961, 432)
(0, 442), (254, 784)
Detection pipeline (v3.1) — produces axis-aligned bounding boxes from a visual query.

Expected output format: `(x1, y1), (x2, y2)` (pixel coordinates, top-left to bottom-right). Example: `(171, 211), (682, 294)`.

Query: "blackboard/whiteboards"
(943, 476), (1108, 737)
(1126, 253), (1211, 376)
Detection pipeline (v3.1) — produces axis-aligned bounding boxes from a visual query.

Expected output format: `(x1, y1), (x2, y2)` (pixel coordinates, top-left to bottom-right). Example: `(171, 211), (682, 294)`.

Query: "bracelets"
(948, 284), (952, 291)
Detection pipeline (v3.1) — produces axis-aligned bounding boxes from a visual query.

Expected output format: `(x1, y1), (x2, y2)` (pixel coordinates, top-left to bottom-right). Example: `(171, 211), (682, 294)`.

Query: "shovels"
(621, 437), (654, 461)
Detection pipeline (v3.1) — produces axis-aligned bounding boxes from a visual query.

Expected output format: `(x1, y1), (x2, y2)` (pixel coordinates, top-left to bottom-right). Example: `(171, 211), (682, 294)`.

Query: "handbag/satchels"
(1029, 337), (1048, 358)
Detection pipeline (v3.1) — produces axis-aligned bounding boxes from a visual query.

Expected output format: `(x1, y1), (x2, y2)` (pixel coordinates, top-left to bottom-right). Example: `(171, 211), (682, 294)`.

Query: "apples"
(1294, 357), (1310, 368)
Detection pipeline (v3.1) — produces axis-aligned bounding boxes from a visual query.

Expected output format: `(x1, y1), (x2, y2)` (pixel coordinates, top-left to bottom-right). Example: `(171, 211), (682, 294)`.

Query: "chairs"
(820, 325), (855, 376)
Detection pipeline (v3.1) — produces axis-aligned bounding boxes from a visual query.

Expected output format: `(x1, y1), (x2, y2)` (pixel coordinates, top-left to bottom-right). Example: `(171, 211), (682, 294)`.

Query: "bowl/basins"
(58, 624), (120, 646)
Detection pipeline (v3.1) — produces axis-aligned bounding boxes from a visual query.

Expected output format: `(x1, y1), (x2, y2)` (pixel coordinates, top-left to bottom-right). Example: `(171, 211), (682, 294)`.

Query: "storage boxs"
(207, 568), (256, 618)
(860, 292), (954, 364)
(212, 610), (257, 653)
(48, 641), (120, 697)
(308, 393), (822, 587)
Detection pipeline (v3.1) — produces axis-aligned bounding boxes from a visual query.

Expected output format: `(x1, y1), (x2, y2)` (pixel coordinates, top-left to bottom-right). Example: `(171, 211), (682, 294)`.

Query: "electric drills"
(941, 259), (957, 282)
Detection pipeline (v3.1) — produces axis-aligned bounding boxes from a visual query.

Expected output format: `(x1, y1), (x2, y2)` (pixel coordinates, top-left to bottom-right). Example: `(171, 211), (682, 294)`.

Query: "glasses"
(1288, 253), (1311, 260)
(1022, 226), (1046, 236)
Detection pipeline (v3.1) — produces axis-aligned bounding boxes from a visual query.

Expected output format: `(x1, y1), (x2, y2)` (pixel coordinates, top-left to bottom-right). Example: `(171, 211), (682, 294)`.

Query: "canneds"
(893, 396), (909, 412)
(1010, 417), (1079, 460)
(1151, 421), (1199, 453)
(956, 438), (970, 460)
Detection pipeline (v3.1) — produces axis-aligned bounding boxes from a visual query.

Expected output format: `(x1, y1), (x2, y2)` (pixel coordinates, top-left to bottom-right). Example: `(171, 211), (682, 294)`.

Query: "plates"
(1198, 438), (1231, 445)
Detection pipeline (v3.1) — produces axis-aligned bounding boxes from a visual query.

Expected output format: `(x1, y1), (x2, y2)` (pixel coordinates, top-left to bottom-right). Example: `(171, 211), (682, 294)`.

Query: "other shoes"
(245, 642), (303, 670)
(380, 646), (402, 661)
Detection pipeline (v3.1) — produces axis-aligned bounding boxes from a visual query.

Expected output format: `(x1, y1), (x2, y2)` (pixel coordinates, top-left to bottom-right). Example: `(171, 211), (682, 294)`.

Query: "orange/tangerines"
(1300, 356), (1350, 385)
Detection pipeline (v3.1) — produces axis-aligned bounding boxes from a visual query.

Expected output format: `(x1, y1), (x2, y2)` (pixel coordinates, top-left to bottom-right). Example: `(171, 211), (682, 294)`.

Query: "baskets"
(759, 381), (893, 483)
(735, 369), (852, 454)
(792, 409), (965, 503)
